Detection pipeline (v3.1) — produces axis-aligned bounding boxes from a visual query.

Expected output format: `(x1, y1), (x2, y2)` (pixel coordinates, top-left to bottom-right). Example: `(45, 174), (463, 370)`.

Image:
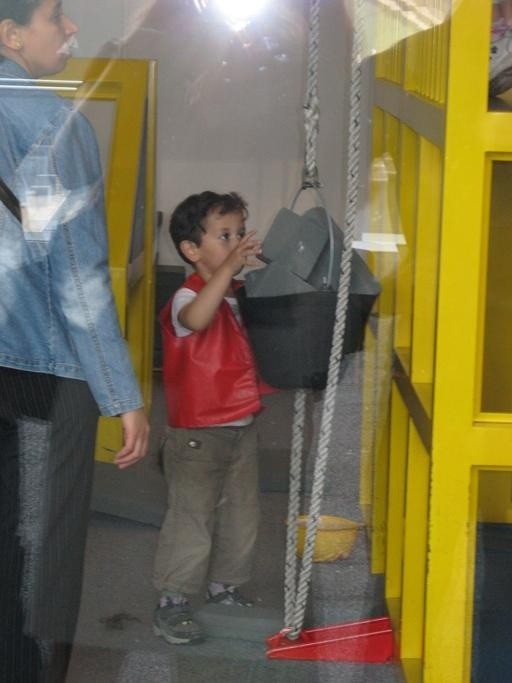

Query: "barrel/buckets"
(233, 187), (381, 389)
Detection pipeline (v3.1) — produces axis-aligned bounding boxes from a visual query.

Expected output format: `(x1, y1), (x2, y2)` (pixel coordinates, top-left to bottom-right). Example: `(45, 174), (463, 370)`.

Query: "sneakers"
(205, 582), (252, 609)
(153, 602), (204, 647)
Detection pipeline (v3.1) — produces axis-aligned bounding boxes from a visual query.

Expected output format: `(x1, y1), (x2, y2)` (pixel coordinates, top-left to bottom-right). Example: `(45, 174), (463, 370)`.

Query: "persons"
(147, 188), (263, 645)
(1, 1), (152, 682)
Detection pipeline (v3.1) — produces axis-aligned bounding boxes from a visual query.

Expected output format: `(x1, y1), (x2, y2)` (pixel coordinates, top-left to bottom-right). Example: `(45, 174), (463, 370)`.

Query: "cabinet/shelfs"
(360, 0), (512, 682)
